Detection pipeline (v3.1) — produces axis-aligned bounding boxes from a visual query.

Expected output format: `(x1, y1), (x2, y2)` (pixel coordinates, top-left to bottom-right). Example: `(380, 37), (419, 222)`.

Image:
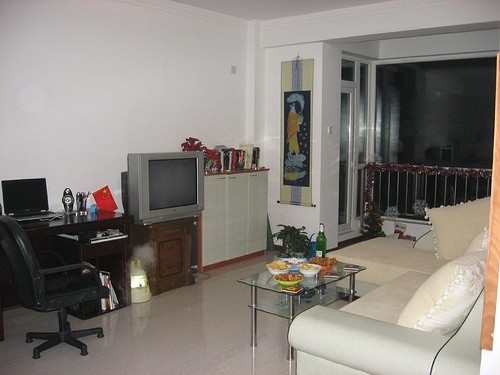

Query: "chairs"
(0, 215), (110, 359)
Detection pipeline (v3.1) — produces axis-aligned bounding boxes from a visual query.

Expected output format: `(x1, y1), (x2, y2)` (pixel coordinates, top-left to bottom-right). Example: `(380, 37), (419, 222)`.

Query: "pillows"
(396, 225), (489, 335)
(423, 195), (491, 259)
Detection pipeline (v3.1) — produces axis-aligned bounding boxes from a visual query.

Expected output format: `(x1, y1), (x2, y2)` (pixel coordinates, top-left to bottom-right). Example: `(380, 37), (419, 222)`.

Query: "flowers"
(181, 137), (206, 157)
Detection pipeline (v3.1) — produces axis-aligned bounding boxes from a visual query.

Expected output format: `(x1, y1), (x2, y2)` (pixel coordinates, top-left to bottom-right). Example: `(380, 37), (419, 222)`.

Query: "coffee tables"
(237, 262), (366, 361)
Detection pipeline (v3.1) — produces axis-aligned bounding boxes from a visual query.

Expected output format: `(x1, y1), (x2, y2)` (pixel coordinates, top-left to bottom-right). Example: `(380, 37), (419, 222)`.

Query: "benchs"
(326, 228), (439, 296)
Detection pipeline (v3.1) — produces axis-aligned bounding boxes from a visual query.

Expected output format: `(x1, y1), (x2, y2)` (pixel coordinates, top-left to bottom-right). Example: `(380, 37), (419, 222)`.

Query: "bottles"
(316, 223), (327, 258)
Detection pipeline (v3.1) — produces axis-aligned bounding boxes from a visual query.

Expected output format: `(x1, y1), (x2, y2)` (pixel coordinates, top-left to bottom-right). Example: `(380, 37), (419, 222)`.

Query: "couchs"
(286, 271), (489, 375)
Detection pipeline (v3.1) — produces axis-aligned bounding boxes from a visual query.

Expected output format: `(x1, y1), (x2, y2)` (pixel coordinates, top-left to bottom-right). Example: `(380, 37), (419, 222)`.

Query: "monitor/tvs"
(121, 151), (204, 226)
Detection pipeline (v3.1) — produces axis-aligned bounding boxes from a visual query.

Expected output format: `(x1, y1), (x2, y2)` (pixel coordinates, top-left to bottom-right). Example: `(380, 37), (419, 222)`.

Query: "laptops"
(0, 178), (62, 221)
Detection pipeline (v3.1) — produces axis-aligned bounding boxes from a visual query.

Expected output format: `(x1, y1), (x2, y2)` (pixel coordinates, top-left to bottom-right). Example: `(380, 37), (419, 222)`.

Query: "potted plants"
(270, 222), (309, 258)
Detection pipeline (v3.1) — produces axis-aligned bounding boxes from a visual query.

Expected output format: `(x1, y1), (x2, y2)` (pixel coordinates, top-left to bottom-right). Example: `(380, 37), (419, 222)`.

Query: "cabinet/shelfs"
(195, 169), (270, 272)
(130, 215), (197, 297)
(0, 210), (135, 341)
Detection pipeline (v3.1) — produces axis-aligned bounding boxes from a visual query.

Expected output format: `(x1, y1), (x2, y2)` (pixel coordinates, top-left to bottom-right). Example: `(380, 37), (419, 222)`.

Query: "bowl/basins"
(299, 264), (321, 277)
(268, 265), (291, 275)
(322, 266), (332, 270)
(273, 275), (304, 286)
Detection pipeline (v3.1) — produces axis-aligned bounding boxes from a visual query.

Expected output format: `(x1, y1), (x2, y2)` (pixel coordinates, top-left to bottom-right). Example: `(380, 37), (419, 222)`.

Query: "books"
(99, 271), (119, 310)
(216, 148), (245, 171)
(240, 144), (260, 169)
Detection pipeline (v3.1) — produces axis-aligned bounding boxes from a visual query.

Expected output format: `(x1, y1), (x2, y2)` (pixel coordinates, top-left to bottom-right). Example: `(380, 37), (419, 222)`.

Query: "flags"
(92, 186), (118, 212)
